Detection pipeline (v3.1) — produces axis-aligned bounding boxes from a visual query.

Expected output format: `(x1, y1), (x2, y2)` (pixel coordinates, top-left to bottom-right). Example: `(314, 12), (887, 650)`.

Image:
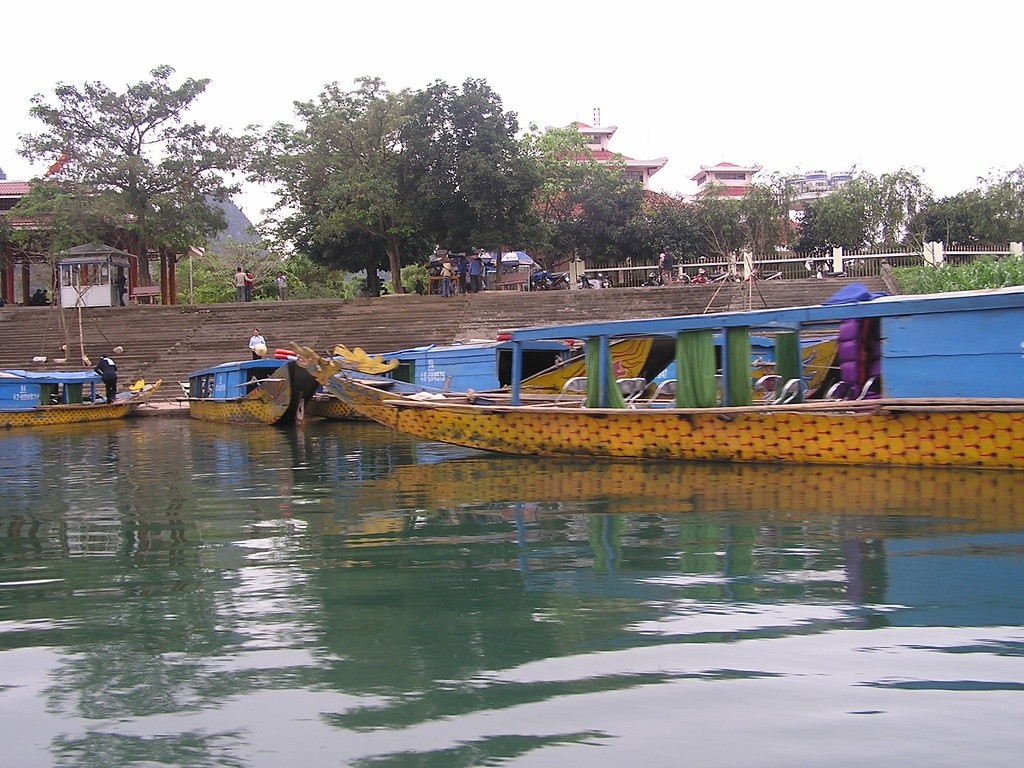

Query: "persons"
(234, 267), (254, 302)
(276, 271), (288, 301)
(531, 266), (553, 291)
(816, 262), (830, 280)
(415, 255), (486, 298)
(0, 289), (50, 307)
(659, 245), (673, 286)
(566, 339), (574, 349)
(94, 355), (118, 404)
(250, 328), (267, 360)
(116, 273), (128, 306)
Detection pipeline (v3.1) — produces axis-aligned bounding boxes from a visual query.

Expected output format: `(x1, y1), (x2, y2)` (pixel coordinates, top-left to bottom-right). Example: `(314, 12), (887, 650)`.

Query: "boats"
(171, 284), (1022, 470)
(0, 367), (163, 427)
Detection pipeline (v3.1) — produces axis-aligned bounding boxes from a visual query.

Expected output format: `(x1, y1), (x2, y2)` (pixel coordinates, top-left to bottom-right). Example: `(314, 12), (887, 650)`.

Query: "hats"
(255, 343), (267, 357)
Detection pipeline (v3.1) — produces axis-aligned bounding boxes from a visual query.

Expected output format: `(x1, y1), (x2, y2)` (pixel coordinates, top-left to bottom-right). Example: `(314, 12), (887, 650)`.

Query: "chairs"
(550, 375), (880, 412)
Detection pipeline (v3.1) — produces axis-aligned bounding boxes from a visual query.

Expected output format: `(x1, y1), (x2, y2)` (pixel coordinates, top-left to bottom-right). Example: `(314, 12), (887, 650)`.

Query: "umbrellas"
(500, 251), (534, 264)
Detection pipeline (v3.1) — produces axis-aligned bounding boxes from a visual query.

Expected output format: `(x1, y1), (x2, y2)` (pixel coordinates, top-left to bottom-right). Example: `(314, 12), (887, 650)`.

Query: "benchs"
(495, 272), (529, 291)
(129, 286), (161, 305)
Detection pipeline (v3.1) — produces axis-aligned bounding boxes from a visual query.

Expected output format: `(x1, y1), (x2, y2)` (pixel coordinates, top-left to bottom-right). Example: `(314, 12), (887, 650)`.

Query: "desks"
(428, 276), (460, 296)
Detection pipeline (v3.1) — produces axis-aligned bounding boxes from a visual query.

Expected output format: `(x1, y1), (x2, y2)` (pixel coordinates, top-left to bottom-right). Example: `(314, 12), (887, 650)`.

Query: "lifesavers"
(274, 348), (298, 360)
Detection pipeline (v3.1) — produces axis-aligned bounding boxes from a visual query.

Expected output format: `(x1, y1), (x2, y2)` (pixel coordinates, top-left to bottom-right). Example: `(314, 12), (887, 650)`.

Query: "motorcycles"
(642, 265), (783, 287)
(808, 259), (849, 279)
(533, 269), (613, 290)
